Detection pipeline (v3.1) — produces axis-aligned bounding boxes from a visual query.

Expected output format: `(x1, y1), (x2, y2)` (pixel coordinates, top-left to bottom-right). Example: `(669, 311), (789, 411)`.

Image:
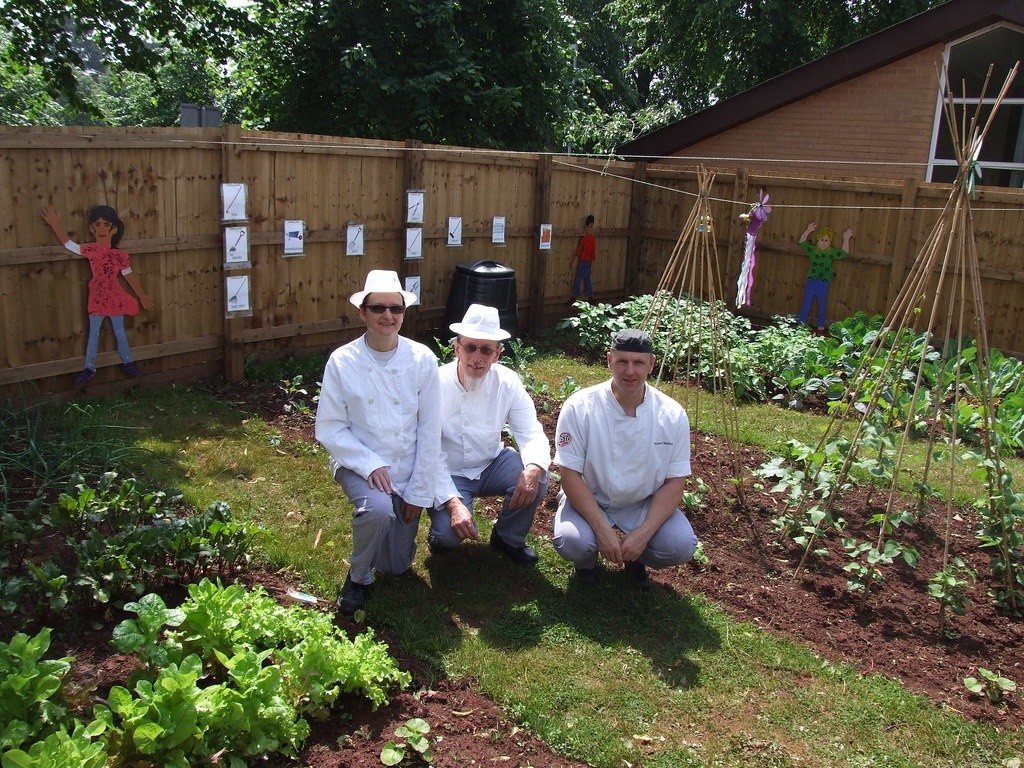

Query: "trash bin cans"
(444, 258), (519, 350)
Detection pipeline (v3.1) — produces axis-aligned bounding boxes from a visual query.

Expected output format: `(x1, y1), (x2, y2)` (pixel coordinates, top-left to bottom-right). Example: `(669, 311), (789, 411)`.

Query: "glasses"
(364, 305), (405, 314)
(457, 340), (499, 355)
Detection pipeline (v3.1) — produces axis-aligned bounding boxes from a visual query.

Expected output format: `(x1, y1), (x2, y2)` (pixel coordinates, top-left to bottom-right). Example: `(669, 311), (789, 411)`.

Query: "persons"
(314, 269), (441, 620)
(553, 330), (698, 584)
(425, 302), (550, 576)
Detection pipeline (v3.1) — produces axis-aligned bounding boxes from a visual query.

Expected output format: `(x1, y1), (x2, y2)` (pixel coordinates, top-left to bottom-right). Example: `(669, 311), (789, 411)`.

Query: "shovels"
(228, 230), (244, 255)
(348, 228), (362, 250)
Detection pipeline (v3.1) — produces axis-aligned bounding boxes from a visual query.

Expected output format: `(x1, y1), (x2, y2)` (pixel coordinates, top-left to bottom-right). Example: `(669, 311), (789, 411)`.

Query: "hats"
(449, 304), (511, 341)
(611, 328), (652, 352)
(350, 269), (417, 310)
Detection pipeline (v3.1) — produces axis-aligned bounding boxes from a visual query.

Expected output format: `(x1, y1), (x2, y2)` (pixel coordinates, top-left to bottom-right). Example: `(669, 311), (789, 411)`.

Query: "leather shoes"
(336, 567), (374, 613)
(428, 533), (446, 553)
(491, 525), (539, 564)
(624, 556), (652, 590)
(577, 563), (600, 583)
